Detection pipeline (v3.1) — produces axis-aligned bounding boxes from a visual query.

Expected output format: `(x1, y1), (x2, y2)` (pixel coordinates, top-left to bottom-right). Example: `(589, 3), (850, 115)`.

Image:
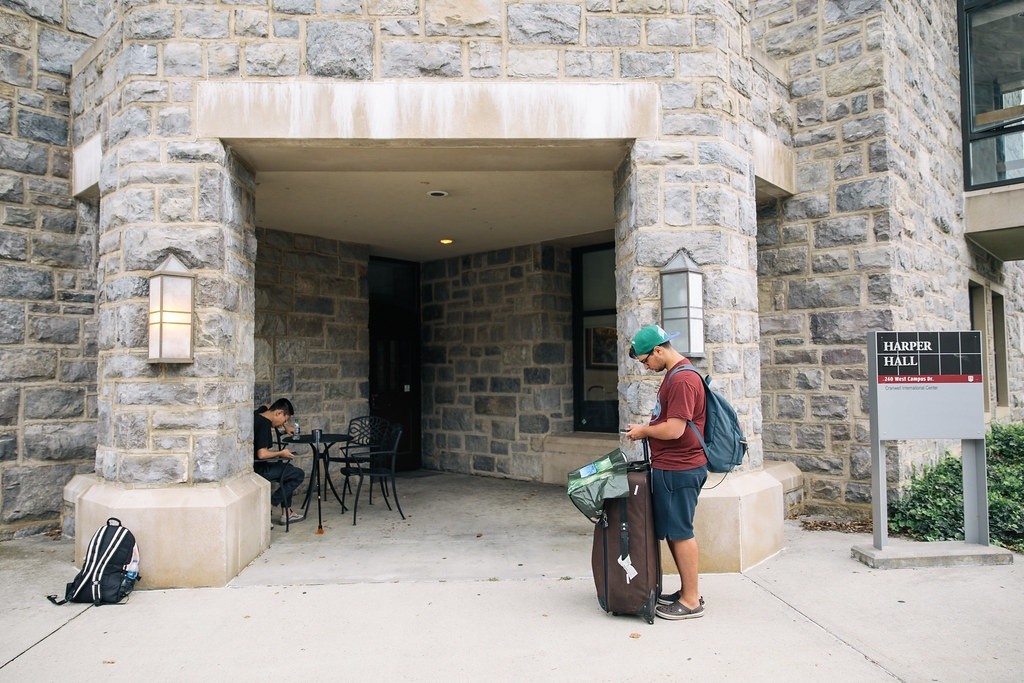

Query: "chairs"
(324, 416), (391, 509)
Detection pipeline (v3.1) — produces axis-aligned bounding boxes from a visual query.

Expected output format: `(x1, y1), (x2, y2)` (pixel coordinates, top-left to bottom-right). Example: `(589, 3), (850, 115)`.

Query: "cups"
(312, 429), (322, 441)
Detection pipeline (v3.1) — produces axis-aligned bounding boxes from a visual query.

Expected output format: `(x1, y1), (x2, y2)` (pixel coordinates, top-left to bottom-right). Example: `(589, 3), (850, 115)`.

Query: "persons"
(254, 398), (305, 529)
(625, 324), (707, 620)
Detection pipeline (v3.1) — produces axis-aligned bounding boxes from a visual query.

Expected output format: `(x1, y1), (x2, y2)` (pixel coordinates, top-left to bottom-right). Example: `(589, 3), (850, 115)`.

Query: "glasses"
(641, 351), (653, 364)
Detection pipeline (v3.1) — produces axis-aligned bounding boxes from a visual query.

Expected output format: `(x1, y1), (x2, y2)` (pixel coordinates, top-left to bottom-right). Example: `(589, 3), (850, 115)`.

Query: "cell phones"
(292, 451), (299, 455)
(620, 429), (631, 434)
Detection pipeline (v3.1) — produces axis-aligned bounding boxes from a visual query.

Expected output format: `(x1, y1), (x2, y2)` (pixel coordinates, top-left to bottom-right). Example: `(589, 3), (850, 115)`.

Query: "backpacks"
(666, 364), (749, 473)
(47, 517), (142, 607)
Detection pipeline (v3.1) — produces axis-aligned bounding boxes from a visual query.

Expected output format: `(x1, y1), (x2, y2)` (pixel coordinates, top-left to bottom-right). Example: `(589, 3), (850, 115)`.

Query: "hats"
(631, 325), (680, 356)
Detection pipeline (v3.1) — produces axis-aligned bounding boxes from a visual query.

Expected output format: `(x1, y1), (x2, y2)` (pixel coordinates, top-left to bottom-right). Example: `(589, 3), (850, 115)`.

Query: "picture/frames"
(587, 326), (617, 369)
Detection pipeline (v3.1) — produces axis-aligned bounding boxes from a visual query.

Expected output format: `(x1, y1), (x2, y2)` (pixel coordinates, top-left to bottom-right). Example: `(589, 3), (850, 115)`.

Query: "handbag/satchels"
(567, 447), (629, 524)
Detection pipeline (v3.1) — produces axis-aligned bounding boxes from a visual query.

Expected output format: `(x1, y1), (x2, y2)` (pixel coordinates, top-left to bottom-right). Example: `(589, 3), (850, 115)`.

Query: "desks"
(282, 434), (353, 517)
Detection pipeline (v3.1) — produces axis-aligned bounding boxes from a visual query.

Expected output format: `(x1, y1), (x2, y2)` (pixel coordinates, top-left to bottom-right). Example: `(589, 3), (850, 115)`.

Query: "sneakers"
(279, 508), (304, 525)
(658, 591), (705, 609)
(655, 601), (704, 619)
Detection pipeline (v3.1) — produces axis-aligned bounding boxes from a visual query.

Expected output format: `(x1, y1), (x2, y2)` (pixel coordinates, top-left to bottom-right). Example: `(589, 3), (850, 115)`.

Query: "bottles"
(293, 419), (300, 440)
(127, 560), (138, 579)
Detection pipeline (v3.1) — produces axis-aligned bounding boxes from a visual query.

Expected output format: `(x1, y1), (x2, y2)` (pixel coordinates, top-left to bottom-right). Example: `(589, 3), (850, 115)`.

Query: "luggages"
(592, 438), (663, 625)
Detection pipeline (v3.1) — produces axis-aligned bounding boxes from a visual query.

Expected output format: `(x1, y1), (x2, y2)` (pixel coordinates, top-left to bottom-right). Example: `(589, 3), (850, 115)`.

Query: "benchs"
(341, 420), (406, 525)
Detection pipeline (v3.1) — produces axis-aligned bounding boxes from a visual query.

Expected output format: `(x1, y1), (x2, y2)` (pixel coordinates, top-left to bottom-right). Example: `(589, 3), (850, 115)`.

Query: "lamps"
(147, 253), (195, 365)
(660, 250), (706, 358)
